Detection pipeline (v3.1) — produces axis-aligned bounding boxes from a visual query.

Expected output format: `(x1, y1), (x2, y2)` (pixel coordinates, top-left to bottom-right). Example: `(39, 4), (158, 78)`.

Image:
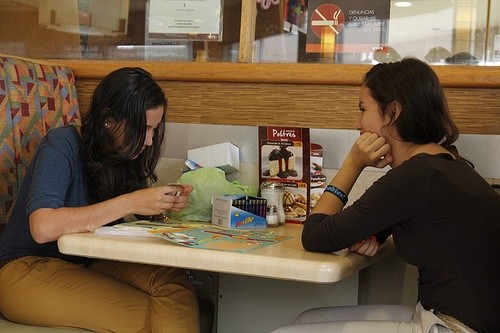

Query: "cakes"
(263, 145), (298, 178)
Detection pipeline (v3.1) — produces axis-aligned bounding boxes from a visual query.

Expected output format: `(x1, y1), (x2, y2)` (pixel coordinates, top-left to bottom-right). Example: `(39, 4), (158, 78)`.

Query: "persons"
(268, 57), (500, 332)
(0, 66), (201, 332)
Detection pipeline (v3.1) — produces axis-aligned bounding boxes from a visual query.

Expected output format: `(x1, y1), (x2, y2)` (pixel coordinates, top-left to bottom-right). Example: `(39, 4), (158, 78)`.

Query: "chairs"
(0, 53), (94, 333)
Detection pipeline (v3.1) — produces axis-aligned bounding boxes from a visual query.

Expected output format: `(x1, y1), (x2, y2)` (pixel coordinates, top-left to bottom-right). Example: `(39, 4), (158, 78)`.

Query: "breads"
(282, 190), (307, 218)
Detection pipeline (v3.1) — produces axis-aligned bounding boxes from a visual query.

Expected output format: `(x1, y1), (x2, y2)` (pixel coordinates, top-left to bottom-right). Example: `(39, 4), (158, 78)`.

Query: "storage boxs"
(187, 140), (240, 175)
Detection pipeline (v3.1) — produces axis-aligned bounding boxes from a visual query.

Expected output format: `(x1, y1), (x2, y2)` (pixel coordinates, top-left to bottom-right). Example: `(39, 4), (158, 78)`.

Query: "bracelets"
(323, 185), (348, 206)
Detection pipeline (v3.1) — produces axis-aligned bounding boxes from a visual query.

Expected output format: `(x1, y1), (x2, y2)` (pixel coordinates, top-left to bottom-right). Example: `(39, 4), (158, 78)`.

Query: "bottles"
(266, 205), (278, 225)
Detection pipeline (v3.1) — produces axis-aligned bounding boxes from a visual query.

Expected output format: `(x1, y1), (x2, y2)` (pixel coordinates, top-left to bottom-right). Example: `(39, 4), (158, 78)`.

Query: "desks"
(57, 220), (396, 333)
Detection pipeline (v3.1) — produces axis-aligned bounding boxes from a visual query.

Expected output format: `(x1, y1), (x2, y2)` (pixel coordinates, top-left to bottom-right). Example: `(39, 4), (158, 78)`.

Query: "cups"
(260, 181), (284, 223)
